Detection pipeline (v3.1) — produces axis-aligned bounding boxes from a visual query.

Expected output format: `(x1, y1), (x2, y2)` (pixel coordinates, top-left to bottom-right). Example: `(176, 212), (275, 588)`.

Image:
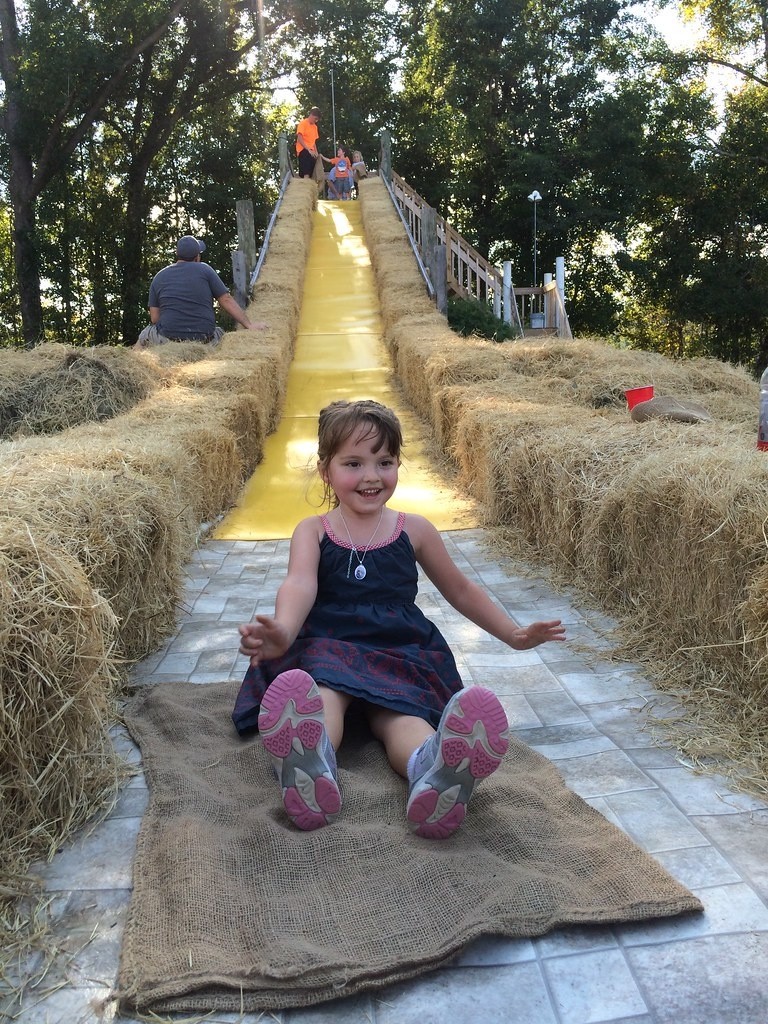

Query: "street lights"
(527, 190), (542, 314)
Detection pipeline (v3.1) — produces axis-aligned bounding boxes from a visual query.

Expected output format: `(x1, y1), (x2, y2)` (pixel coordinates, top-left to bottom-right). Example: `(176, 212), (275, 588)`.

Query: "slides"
(4, 173), (768, 1016)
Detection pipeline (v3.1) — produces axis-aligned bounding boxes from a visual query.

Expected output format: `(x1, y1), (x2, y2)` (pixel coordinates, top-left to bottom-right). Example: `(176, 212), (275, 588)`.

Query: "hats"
(177, 236), (206, 258)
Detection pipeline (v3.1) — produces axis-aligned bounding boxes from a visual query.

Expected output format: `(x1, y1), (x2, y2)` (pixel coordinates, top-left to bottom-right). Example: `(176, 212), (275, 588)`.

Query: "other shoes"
(408, 686), (509, 838)
(257, 669), (342, 831)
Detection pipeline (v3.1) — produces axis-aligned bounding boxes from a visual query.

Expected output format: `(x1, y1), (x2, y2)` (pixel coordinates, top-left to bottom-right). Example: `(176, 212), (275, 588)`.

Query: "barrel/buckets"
(624, 385), (654, 413)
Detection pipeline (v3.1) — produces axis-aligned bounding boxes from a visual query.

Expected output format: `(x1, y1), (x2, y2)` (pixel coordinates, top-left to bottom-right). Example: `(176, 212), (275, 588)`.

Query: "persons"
(319, 145), (367, 201)
(136, 235), (269, 347)
(231, 400), (566, 838)
(296, 106), (322, 178)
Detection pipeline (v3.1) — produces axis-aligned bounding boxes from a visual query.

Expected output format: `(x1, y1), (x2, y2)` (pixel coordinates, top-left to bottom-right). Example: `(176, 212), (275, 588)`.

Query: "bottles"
(757, 366), (768, 451)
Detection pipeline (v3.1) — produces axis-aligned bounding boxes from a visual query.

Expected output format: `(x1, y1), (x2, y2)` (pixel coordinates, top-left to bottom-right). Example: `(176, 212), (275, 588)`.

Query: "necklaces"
(338, 504), (383, 580)
